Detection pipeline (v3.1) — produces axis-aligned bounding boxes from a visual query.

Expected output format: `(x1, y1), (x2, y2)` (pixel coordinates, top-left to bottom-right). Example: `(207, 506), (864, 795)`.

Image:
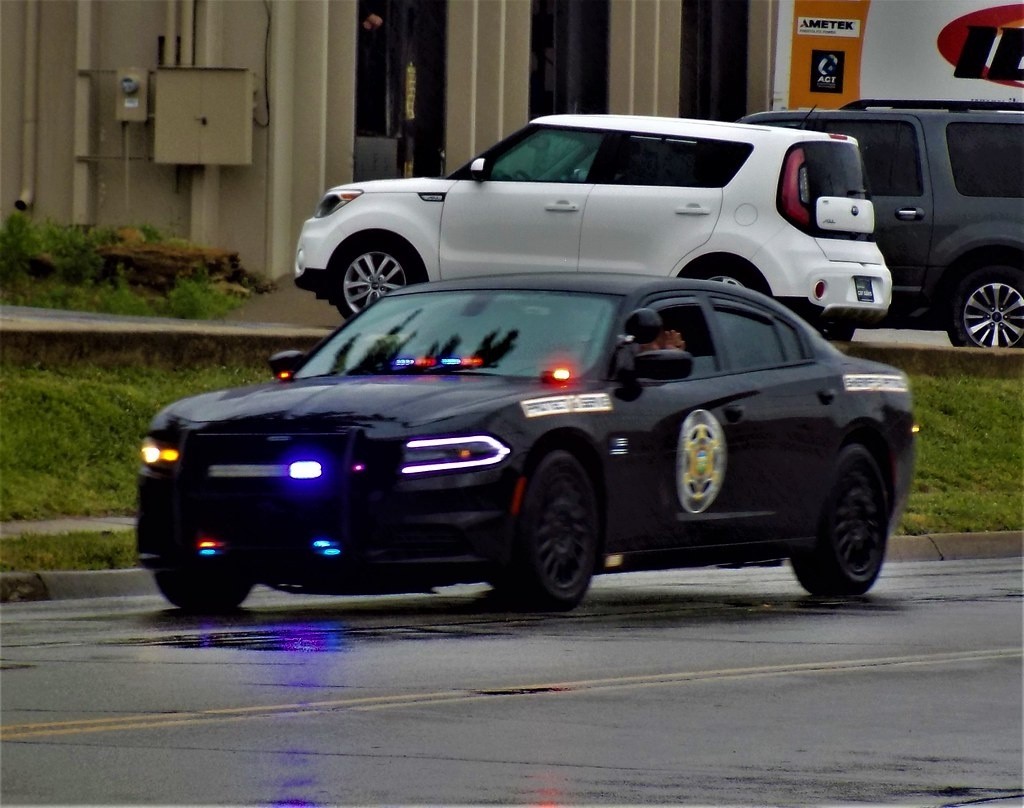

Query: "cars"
(133, 271), (920, 614)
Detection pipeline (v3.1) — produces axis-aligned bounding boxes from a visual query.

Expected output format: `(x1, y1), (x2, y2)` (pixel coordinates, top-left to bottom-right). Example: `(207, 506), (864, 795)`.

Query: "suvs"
(730, 97), (1024, 348)
(290, 112), (893, 346)
